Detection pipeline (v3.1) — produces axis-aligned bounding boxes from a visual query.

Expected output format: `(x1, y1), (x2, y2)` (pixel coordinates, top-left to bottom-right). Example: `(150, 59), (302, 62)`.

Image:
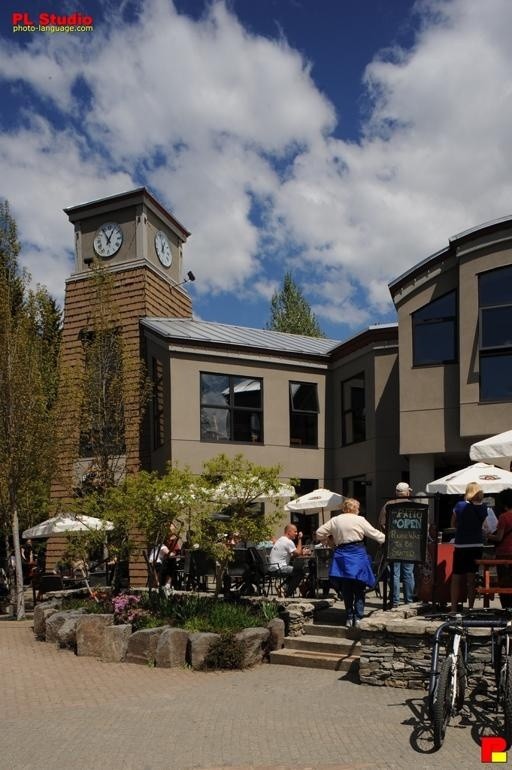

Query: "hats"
(395, 482), (414, 491)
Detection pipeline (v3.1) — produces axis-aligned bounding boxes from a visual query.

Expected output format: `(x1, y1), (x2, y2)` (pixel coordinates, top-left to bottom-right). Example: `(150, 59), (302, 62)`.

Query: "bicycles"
(489, 606), (512, 750)
(421, 610), (483, 751)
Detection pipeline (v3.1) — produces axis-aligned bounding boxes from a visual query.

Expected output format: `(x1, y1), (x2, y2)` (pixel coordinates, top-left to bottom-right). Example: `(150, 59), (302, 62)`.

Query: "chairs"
(141, 546), (388, 599)
(32, 572), (64, 607)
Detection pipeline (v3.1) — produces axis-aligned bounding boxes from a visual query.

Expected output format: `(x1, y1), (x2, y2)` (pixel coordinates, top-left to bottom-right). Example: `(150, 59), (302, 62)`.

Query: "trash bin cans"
(90, 572), (111, 588)
(163, 556), (187, 591)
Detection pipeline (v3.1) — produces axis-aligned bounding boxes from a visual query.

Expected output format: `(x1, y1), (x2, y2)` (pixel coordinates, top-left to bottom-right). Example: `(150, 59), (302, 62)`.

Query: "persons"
(377, 482), (413, 608)
(315, 498), (386, 627)
(269, 526), (308, 597)
(451, 481), (512, 613)
(147, 523), (275, 593)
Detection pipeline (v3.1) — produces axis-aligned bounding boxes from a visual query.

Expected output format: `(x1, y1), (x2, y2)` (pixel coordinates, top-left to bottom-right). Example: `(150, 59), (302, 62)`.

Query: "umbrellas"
(425, 429), (512, 494)
(285, 487), (346, 525)
(157, 474), (295, 542)
(22, 511), (117, 539)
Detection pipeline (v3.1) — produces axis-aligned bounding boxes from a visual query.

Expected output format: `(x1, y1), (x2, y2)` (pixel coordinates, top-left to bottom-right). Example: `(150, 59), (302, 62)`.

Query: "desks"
(63, 576), (86, 587)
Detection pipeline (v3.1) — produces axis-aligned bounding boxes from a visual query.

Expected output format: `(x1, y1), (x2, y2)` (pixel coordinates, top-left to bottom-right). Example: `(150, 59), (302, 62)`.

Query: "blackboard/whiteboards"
(386, 502), (428, 563)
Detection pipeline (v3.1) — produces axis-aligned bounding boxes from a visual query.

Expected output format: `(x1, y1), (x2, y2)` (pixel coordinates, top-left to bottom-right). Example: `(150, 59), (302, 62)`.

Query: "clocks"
(153, 229), (173, 268)
(93, 221), (124, 257)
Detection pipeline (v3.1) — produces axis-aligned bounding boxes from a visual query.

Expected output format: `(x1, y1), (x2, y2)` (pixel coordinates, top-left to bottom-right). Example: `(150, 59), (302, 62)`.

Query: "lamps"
(169, 271), (195, 290)
(83, 257), (105, 281)
